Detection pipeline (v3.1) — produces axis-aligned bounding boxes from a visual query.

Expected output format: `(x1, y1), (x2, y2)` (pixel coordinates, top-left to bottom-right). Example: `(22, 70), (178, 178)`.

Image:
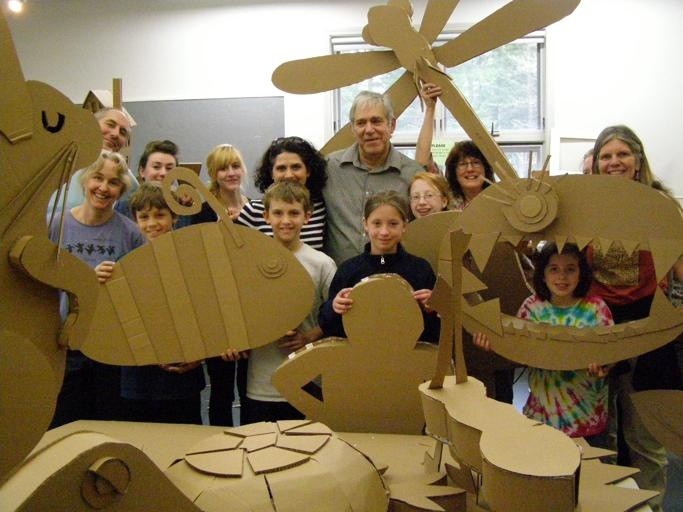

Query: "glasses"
(455, 158), (481, 171)
(271, 136), (304, 146)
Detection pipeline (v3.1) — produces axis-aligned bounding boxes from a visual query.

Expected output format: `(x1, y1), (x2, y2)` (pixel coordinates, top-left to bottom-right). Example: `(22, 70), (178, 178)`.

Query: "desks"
(610, 471), (657, 512)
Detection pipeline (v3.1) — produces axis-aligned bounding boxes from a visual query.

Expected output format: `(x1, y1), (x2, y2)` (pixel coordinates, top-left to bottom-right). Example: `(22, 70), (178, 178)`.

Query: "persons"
(43, 88), (683, 480)
